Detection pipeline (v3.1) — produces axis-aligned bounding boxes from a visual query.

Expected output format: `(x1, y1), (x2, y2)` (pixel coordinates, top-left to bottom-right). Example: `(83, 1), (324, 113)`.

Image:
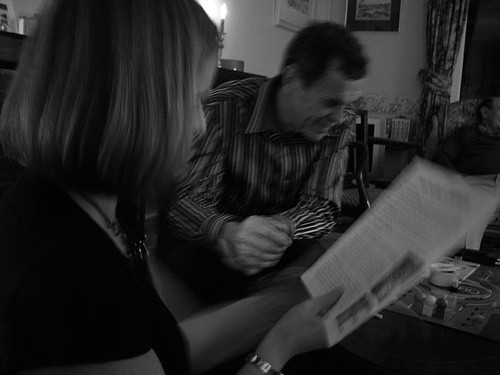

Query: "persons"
(0, 0), (346, 375)
(426, 96), (500, 262)
(148, 19), (369, 320)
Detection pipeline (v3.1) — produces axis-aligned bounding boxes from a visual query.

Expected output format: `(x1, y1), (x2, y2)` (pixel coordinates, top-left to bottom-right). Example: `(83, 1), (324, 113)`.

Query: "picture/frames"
(273, 0), (319, 33)
(346, 0), (401, 32)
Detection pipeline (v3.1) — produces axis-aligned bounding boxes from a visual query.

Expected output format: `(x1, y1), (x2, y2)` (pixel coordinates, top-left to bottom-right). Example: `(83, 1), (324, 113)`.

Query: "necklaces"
(65, 173), (150, 264)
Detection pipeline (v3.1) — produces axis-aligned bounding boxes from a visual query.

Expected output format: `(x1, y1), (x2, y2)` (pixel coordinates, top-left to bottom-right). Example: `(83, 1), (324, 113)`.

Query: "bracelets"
(245, 353), (284, 375)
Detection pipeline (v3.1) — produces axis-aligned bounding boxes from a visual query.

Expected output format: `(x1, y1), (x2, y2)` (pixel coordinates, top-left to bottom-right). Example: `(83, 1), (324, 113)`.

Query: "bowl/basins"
(221, 60), (243, 72)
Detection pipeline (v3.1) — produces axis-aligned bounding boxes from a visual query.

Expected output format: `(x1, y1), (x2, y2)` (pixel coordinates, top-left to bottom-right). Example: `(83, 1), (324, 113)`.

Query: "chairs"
(339, 108), (414, 232)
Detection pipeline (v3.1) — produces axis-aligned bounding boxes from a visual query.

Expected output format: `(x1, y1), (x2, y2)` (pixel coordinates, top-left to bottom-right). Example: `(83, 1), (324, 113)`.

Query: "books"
(386, 117), (417, 143)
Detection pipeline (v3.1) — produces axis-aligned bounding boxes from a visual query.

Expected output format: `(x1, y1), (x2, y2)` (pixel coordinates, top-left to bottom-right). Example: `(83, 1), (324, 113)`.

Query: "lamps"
(215, 3), (227, 69)
